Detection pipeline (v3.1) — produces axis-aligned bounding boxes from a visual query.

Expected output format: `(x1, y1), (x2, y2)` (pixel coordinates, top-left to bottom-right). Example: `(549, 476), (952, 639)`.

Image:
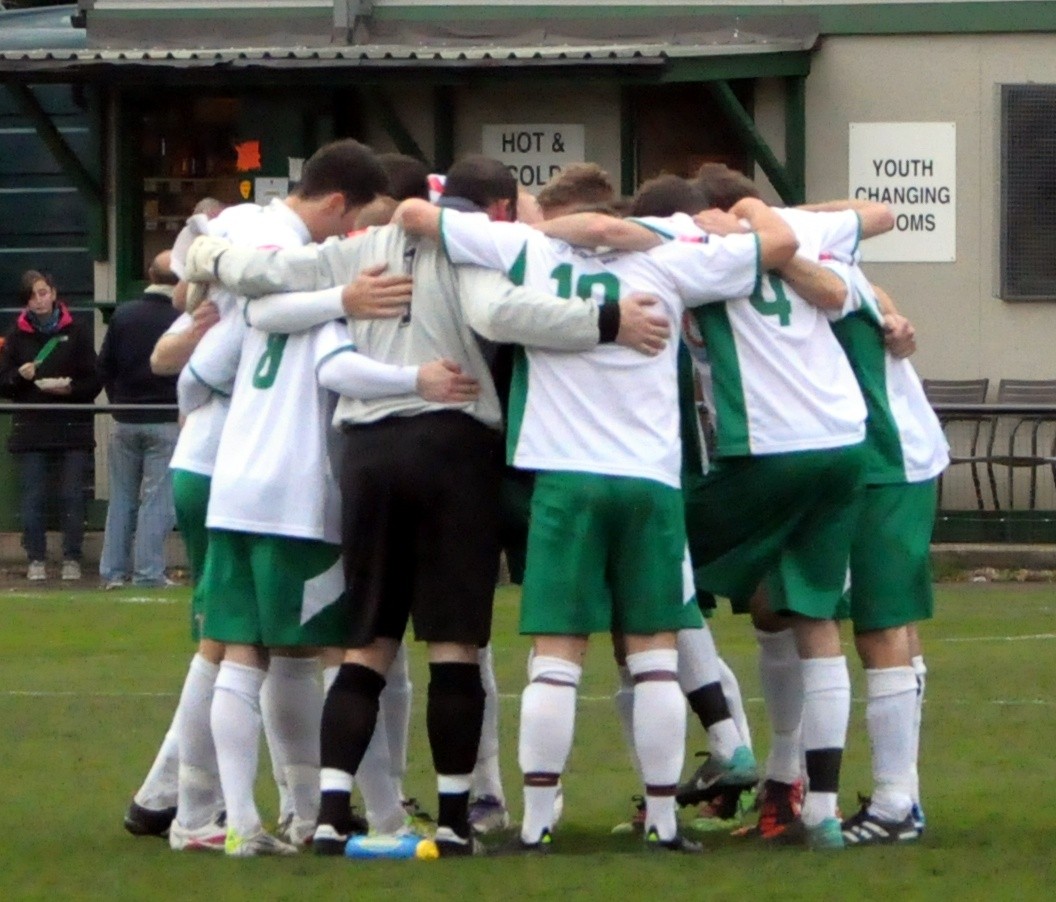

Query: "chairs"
(920, 378), (1055, 540)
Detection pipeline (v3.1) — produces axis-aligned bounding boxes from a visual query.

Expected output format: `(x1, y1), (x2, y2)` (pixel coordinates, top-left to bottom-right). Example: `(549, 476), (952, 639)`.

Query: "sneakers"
(313, 815), (369, 855)
(369, 813), (436, 842)
(277, 807), (315, 847)
(753, 745), (806, 844)
(61, 559), (81, 580)
(676, 750), (730, 795)
(610, 795), (683, 835)
(26, 560), (46, 580)
(399, 796), (433, 834)
(687, 781), (760, 833)
(225, 827), (299, 858)
(486, 826), (558, 857)
(434, 826), (496, 858)
(468, 794), (510, 833)
(123, 800), (227, 838)
(642, 824), (706, 854)
(796, 806), (847, 851)
(169, 809), (226, 852)
(840, 790), (926, 848)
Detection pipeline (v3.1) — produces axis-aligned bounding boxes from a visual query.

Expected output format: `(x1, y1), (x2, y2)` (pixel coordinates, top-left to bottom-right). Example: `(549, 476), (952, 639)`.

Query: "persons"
(123, 140), (952, 859)
(98, 251), (181, 588)
(0, 269), (103, 583)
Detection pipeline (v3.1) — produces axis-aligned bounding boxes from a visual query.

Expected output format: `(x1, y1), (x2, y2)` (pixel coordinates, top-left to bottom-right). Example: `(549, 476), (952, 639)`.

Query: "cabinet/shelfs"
(143, 176), (239, 223)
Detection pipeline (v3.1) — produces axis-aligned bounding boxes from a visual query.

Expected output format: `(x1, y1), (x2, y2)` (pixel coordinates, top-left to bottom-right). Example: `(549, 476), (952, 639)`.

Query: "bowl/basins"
(34, 378), (72, 389)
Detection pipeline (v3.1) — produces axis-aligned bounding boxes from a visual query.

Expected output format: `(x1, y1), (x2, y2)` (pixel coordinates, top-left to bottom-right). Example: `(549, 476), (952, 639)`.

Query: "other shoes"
(98, 579), (124, 590)
(165, 579), (182, 588)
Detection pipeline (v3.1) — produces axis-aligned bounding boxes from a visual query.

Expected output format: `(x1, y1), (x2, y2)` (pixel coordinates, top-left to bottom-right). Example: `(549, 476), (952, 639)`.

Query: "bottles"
(344, 835), (440, 860)
(148, 124), (207, 178)
